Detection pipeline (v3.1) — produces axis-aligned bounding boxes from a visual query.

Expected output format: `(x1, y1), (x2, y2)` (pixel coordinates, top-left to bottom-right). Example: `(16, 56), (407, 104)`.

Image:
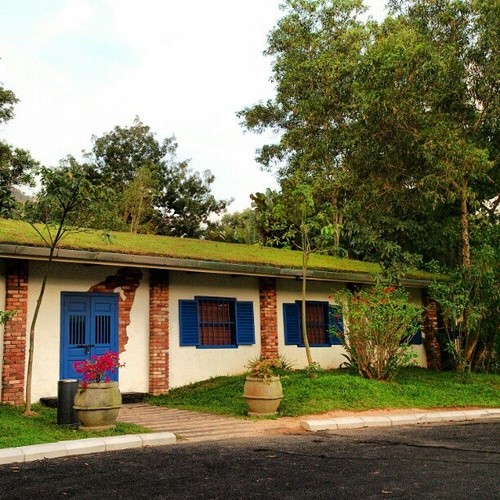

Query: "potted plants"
(240, 353), (297, 416)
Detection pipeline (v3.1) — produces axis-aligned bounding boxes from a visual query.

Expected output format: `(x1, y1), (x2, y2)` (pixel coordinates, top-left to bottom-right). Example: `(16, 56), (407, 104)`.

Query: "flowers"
(73, 349), (125, 390)
